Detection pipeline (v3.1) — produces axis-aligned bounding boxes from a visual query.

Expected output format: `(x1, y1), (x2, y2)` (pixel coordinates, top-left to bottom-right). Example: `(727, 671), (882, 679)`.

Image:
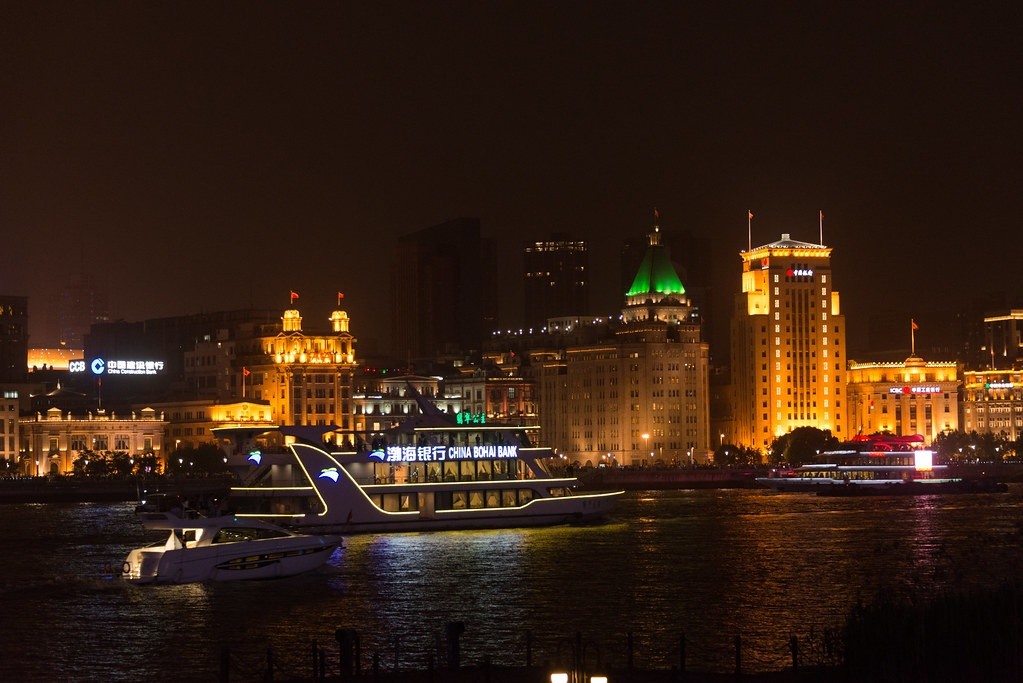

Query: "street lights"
(720, 434), (726, 447)
(175, 439), (181, 450)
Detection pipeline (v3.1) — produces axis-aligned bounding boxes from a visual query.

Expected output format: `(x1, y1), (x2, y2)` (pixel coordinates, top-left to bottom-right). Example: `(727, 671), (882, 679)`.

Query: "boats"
(121, 518), (344, 584)
(756, 441), (1008, 493)
(211, 375), (625, 532)
